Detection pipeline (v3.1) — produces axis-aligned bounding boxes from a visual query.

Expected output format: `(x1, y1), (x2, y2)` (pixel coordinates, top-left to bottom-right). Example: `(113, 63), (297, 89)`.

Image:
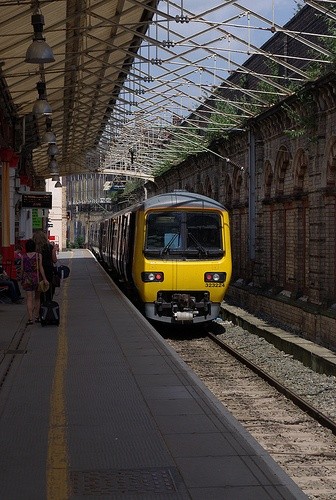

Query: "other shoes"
(34, 318), (40, 322)
(13, 300), (21, 304)
(17, 296), (24, 299)
(26, 320), (33, 324)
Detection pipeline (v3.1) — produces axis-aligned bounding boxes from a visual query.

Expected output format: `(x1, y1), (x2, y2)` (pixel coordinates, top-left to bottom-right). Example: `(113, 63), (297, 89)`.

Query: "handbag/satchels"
(55, 275), (60, 287)
(38, 280), (49, 293)
(54, 265), (70, 279)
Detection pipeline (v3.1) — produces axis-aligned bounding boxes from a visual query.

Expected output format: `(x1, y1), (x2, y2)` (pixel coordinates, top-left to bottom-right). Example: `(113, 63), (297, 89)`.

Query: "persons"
(14, 240), (23, 266)
(21, 238), (49, 324)
(34, 232), (57, 302)
(0, 267), (24, 304)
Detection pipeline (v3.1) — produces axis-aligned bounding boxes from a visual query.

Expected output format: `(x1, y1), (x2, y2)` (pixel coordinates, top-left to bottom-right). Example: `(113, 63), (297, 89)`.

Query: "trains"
(98, 179), (232, 341)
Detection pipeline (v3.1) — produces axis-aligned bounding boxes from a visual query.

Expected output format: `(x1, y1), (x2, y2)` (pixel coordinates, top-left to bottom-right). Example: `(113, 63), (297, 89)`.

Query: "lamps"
(24, 3), (62, 187)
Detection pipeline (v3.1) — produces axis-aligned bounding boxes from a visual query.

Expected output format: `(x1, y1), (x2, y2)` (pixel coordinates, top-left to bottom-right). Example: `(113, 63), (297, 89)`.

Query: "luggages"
(39, 283), (60, 327)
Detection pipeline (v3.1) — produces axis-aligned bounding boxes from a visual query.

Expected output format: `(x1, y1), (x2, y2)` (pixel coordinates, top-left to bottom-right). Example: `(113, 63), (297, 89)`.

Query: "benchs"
(0, 286), (11, 303)
